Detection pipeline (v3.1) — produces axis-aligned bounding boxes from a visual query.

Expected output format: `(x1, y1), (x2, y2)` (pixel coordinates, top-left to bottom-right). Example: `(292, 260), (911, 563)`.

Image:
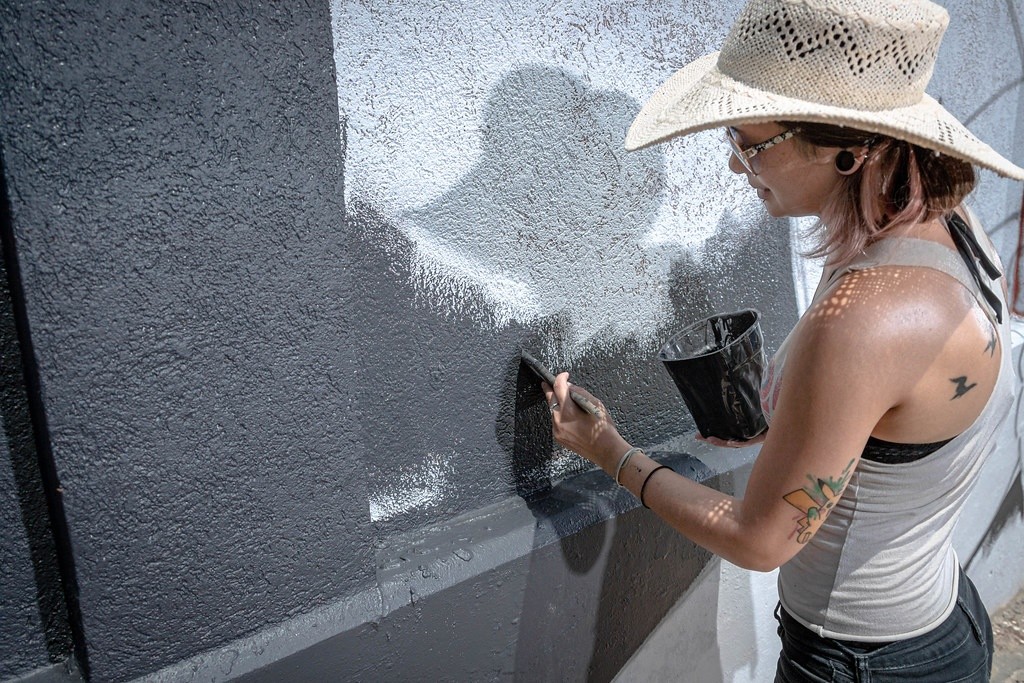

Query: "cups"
(655, 308), (768, 442)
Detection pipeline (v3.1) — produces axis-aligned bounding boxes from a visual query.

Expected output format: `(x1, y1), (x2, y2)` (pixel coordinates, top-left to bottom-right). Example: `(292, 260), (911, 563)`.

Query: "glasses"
(725, 126), (801, 177)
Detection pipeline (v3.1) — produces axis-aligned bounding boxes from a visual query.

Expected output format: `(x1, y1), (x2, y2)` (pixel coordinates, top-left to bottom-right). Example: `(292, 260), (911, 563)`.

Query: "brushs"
(522, 349), (605, 419)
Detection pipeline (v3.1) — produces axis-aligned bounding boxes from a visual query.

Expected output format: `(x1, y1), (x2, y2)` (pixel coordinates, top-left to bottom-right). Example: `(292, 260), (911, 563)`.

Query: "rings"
(549, 402), (558, 415)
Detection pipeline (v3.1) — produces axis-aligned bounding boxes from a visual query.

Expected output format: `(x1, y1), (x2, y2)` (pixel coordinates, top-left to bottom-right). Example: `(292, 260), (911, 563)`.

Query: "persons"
(540, 0), (1024, 683)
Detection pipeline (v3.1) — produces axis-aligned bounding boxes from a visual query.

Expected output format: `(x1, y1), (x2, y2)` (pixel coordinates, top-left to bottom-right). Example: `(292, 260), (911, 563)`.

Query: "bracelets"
(640, 465), (674, 509)
(615, 447), (644, 488)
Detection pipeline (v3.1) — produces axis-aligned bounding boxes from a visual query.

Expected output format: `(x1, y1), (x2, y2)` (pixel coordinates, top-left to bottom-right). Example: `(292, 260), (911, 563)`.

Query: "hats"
(625, 0), (1024, 182)
